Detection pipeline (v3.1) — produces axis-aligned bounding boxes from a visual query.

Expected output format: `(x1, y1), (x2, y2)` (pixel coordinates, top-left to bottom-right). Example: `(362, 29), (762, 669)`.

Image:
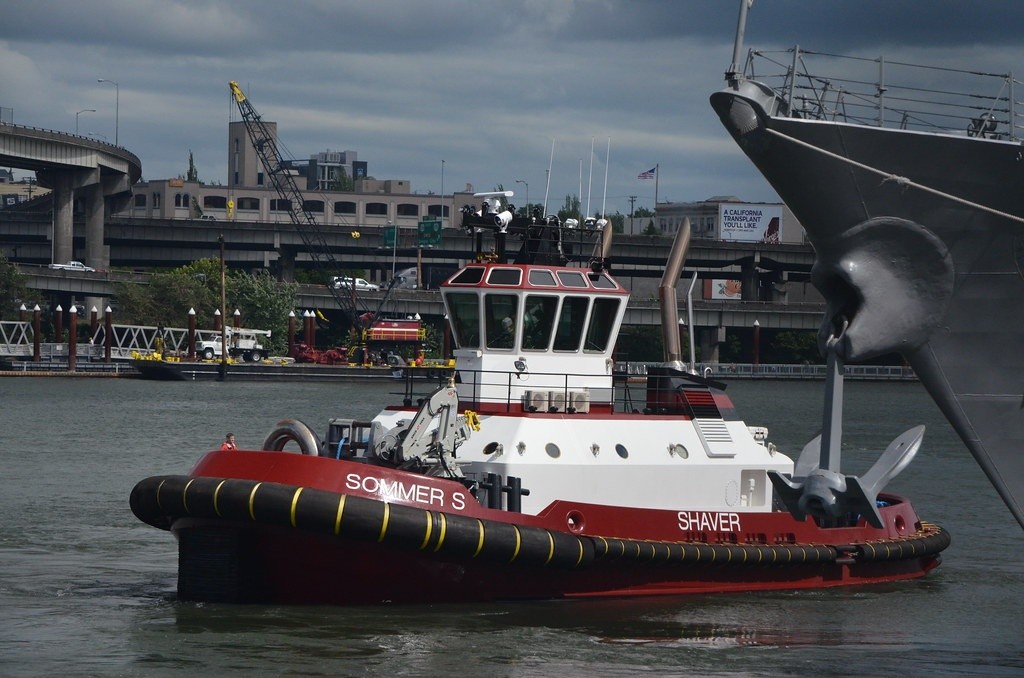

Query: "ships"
(129, 77), (458, 386)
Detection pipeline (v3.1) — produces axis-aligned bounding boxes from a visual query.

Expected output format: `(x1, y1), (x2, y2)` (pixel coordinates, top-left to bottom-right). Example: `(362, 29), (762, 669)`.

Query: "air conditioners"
(524, 389), (549, 413)
(570, 391), (591, 414)
(548, 391), (571, 414)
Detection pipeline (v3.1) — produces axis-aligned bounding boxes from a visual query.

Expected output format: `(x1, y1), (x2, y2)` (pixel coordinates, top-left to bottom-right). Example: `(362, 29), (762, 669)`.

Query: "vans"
(384, 266), (417, 291)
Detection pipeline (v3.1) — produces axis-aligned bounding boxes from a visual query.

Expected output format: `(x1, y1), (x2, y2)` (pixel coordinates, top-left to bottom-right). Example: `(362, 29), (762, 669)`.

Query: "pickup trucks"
(50, 261), (95, 273)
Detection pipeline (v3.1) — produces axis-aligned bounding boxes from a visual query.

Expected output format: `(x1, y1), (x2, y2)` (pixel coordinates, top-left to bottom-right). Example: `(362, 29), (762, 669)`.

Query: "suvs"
(329, 273), (383, 293)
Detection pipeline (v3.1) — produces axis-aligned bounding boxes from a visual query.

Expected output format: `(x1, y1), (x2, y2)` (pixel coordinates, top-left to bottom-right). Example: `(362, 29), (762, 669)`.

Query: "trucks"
(195, 330), (265, 362)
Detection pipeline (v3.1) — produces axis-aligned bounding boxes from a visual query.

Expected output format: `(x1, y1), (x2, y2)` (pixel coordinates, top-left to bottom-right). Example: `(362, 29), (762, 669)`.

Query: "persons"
(355, 346), (424, 369)
(221, 432), (239, 451)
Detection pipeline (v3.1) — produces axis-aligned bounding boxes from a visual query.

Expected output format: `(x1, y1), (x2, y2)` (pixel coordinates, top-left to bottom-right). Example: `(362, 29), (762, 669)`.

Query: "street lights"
(74, 108), (95, 139)
(441, 159), (445, 230)
(88, 131), (107, 142)
(97, 78), (119, 146)
(516, 178), (531, 218)
(387, 199), (398, 278)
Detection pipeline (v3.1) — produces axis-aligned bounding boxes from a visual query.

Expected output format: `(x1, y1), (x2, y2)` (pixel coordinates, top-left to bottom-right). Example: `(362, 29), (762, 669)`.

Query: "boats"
(129, 189), (955, 605)
(714, 0), (1021, 553)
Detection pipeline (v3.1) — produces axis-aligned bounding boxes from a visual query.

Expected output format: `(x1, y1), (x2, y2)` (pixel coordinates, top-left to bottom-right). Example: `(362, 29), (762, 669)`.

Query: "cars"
(195, 213), (217, 221)
